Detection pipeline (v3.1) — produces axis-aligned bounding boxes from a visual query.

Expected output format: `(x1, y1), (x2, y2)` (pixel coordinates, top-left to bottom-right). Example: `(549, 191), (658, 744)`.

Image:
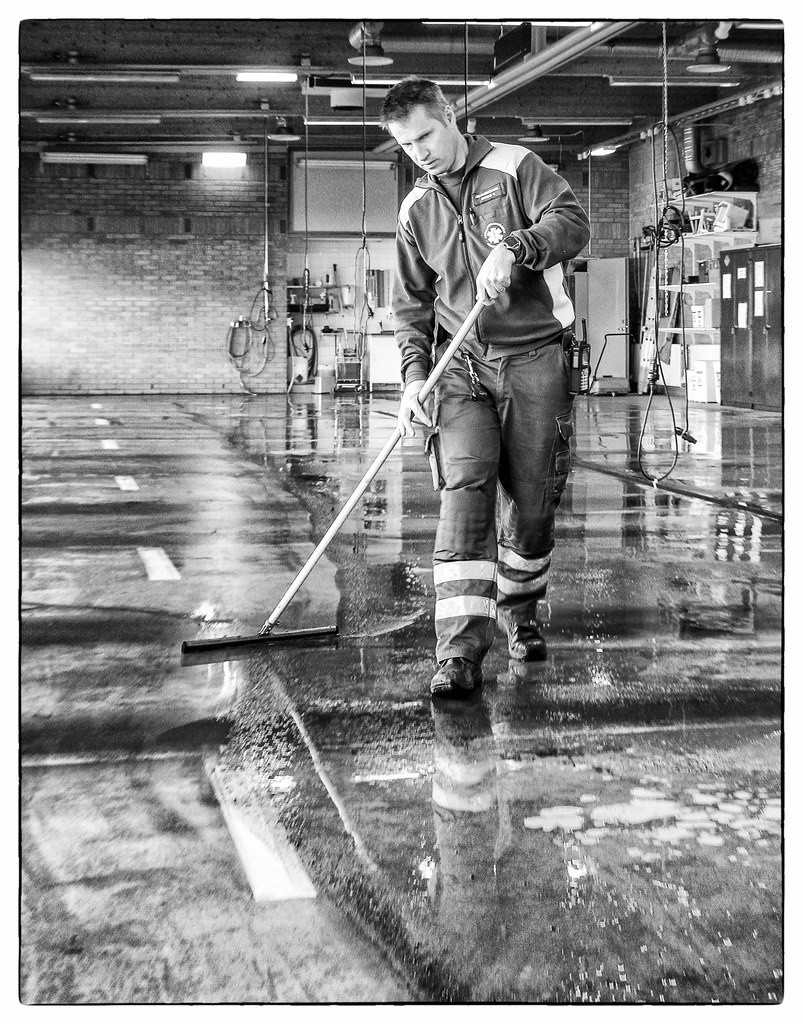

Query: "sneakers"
(430, 657), (484, 692)
(498, 612), (548, 660)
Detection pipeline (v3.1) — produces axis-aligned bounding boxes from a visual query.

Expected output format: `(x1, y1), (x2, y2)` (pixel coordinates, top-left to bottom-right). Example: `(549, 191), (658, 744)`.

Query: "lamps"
(346, 43), (394, 66)
(265, 116), (301, 141)
(517, 124), (549, 142)
(200, 150), (247, 170)
(686, 49), (730, 73)
(590, 145), (616, 156)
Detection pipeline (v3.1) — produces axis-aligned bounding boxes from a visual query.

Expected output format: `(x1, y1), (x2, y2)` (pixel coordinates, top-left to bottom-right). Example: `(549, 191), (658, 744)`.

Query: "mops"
(181, 291), (488, 657)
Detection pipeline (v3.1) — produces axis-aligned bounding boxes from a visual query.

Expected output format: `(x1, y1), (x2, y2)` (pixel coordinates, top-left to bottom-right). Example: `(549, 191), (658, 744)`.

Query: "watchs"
(502, 233), (523, 265)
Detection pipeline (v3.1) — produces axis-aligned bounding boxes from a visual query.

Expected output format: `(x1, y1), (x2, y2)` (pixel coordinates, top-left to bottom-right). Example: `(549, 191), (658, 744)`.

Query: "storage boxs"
(684, 369), (721, 404)
(688, 343), (720, 373)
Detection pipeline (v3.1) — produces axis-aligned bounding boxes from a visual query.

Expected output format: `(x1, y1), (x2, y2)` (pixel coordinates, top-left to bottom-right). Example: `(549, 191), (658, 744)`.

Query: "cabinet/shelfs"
(649, 190), (760, 404)
(719, 247), (753, 409)
(753, 244), (783, 410)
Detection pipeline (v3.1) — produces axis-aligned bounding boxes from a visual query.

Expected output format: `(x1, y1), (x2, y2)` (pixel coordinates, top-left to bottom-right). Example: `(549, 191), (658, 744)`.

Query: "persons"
(378, 76), (590, 694)
